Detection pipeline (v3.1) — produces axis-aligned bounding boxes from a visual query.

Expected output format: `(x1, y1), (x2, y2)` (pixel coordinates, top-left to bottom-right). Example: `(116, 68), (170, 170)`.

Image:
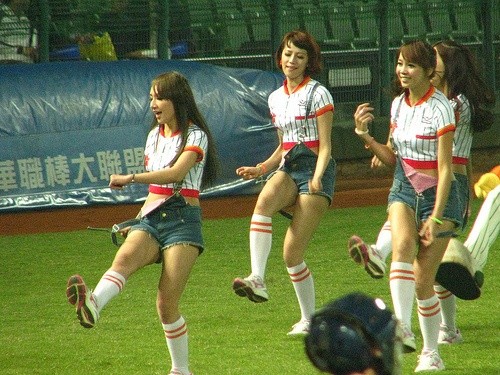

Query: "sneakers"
(286, 319), (310, 335)
(348, 235), (387, 279)
(66, 275), (99, 329)
(233, 273), (268, 304)
(438, 324), (461, 344)
(399, 321), (417, 354)
(414, 347), (446, 372)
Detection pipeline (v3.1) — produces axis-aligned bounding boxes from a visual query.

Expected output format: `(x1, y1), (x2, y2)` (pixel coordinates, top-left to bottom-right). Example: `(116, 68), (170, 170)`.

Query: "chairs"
(24, 0), (500, 66)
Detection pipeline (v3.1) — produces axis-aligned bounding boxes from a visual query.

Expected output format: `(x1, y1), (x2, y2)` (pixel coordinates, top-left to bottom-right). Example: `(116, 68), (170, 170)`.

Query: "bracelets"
(131, 173), (136, 183)
(355, 127), (369, 135)
(365, 136), (374, 150)
(254, 166), (262, 179)
(428, 216), (442, 225)
(256, 163), (267, 175)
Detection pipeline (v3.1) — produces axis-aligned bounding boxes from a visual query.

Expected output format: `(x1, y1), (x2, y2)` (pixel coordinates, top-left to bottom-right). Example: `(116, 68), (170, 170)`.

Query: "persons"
(434, 164), (500, 300)
(348, 40), (473, 344)
(303, 291), (399, 375)
(354, 42), (457, 373)
(68, 71), (219, 375)
(233, 31), (336, 336)
(0, 0), (192, 64)
